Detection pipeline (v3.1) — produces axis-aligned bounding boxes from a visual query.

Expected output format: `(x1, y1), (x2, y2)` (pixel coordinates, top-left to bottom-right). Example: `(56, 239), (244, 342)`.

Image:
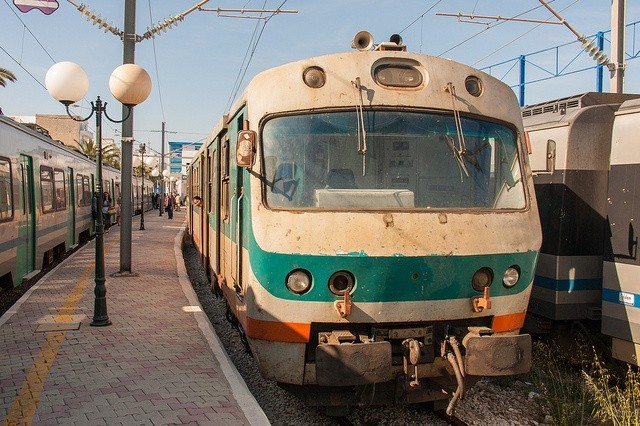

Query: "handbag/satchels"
(108, 206), (117, 216)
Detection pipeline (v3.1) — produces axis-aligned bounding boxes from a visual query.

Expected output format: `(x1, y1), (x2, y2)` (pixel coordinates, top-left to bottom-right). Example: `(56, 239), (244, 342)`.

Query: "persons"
(103, 193), (111, 231)
(191, 195), (204, 207)
(153, 190), (181, 219)
(116, 202), (123, 227)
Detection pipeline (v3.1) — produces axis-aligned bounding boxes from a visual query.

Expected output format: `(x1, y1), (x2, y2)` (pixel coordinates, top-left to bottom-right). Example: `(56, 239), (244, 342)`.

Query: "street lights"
(45, 62), (151, 326)
(152, 169), (170, 217)
(132, 156), (157, 230)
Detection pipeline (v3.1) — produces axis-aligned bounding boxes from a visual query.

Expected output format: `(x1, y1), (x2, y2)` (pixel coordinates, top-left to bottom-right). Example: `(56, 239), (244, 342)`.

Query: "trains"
(0, 114), (154, 288)
(186, 31), (543, 416)
(521, 92), (640, 367)
